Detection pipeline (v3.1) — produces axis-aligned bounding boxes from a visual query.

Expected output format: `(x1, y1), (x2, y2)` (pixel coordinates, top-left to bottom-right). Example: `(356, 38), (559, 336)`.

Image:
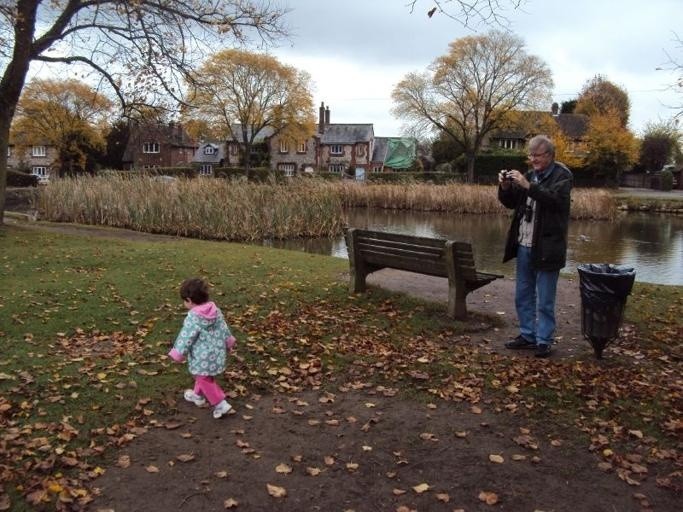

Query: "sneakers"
(184, 389), (206, 406)
(504, 334), (537, 349)
(535, 345), (553, 359)
(213, 399), (232, 419)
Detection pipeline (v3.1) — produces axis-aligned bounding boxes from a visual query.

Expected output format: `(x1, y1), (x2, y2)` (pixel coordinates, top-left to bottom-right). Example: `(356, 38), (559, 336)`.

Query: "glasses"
(526, 152), (548, 158)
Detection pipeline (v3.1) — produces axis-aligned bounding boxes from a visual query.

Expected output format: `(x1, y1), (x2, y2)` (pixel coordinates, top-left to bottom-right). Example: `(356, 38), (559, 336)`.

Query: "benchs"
(341, 226), (504, 321)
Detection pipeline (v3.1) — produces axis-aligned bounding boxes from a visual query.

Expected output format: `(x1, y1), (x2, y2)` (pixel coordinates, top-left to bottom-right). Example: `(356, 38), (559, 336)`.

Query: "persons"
(496, 134), (573, 358)
(166, 276), (237, 422)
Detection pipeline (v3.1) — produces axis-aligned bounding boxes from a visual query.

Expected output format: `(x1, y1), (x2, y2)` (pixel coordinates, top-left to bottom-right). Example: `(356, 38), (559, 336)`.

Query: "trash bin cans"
(577, 263), (635, 359)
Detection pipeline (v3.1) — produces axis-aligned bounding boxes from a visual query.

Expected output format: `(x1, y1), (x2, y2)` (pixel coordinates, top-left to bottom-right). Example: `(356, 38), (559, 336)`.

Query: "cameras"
(502, 171), (512, 179)
